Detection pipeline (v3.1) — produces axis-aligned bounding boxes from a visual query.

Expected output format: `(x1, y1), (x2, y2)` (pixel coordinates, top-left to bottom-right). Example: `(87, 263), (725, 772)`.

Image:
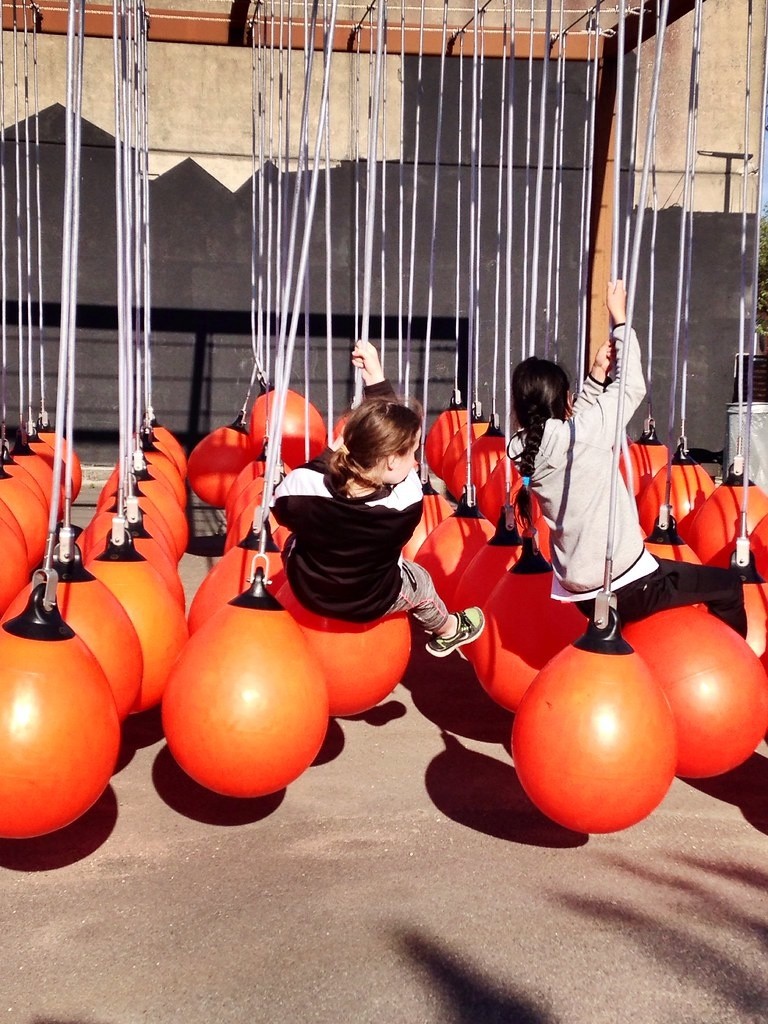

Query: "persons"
(268, 339), (485, 659)
(505, 278), (748, 640)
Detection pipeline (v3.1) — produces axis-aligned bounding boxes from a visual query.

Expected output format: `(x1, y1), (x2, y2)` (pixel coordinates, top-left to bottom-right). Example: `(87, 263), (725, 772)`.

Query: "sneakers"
(425, 606), (485, 658)
